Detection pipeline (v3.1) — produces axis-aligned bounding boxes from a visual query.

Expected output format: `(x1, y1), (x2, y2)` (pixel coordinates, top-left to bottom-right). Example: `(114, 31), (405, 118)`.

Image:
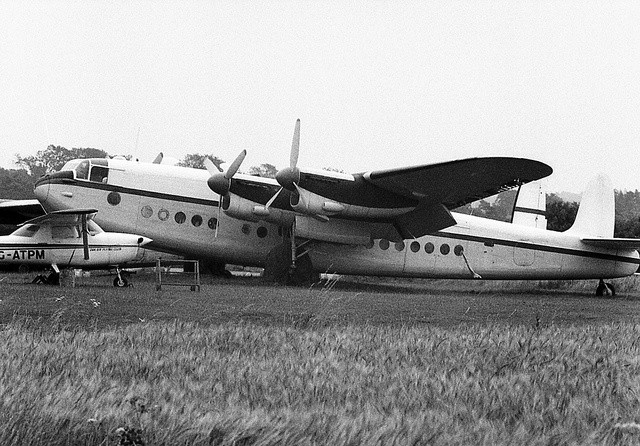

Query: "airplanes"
(32, 117), (640, 297)
(0, 207), (156, 288)
